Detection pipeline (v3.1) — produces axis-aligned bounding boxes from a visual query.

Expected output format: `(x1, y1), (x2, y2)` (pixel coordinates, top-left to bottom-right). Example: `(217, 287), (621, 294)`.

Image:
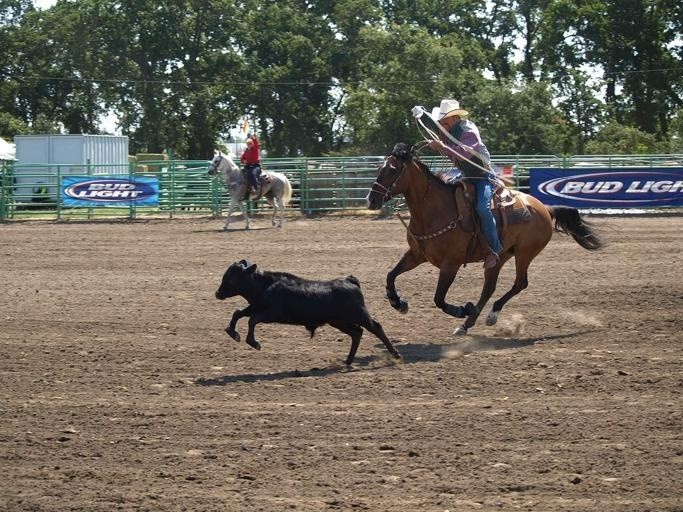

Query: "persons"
(412, 98), (502, 270)
(240, 132), (263, 199)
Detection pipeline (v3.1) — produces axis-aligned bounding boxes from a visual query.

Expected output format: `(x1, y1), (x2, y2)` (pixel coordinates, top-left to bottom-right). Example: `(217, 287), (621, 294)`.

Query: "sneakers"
(483, 249), (504, 269)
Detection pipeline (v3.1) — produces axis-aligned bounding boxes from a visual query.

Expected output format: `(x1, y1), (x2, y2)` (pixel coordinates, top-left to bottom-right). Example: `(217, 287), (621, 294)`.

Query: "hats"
(244, 138), (253, 144)
(432, 98), (469, 121)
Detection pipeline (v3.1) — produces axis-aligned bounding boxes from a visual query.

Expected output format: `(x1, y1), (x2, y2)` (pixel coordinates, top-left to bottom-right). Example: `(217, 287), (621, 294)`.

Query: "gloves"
(411, 106), (423, 120)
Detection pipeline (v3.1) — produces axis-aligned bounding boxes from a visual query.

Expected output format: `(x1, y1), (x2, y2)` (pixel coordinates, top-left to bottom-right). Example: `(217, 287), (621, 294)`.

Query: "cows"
(214, 259), (402, 366)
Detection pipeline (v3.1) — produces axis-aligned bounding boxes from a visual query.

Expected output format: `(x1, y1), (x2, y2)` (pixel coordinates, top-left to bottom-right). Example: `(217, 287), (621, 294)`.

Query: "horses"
(207, 148), (292, 230)
(366, 142), (623, 336)
